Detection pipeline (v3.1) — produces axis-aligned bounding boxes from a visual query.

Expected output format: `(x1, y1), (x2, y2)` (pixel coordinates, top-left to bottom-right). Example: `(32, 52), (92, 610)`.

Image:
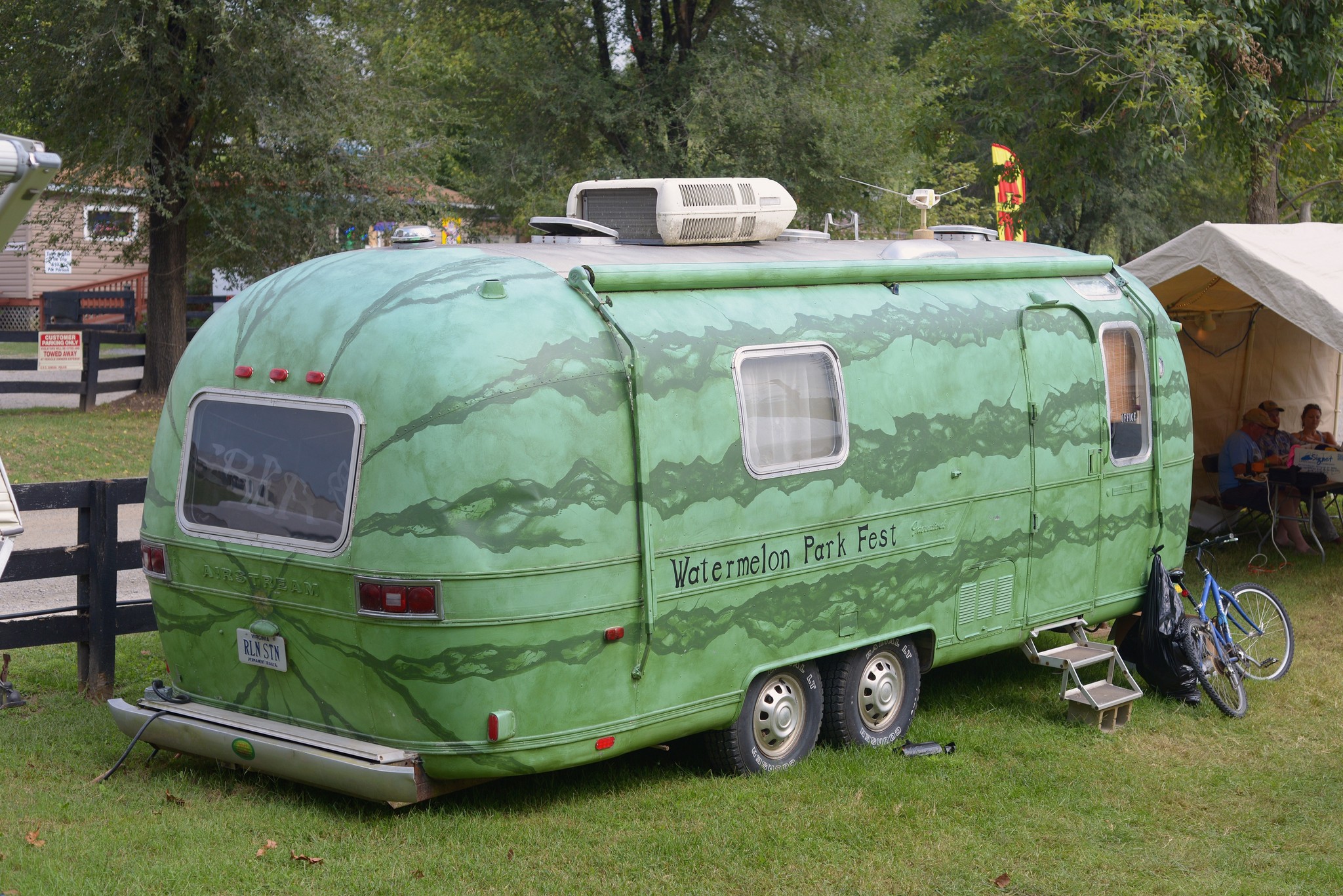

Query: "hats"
(1242, 408), (1277, 429)
(1259, 400), (1285, 414)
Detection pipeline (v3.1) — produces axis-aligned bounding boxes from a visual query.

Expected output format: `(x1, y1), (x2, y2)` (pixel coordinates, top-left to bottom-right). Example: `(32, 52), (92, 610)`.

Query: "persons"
(1218, 409), (1322, 557)
(1257, 401), (1343, 548)
(1290, 404), (1343, 495)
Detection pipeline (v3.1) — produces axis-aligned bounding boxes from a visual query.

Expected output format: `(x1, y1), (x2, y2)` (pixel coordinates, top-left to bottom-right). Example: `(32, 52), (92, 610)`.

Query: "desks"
(1235, 472), (1343, 564)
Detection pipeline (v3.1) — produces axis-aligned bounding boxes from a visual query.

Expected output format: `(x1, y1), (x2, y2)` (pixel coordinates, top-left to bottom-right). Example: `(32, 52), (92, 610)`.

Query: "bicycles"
(1166, 533), (1296, 720)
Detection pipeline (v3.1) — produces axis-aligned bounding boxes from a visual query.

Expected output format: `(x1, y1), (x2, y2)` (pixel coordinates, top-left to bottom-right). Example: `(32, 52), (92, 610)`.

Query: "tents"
(1116, 221), (1343, 503)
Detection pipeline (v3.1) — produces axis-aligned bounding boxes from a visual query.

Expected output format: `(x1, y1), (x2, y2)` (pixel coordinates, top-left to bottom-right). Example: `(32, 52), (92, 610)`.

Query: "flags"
(993, 142), (1026, 242)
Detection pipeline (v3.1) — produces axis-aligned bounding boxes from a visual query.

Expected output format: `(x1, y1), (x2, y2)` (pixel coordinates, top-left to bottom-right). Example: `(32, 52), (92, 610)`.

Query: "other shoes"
(1295, 546), (1322, 557)
(1271, 539), (1296, 548)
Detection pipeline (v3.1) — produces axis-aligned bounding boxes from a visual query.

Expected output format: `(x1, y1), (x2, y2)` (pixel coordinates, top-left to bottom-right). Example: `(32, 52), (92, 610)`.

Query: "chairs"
(1199, 453), (1279, 549)
